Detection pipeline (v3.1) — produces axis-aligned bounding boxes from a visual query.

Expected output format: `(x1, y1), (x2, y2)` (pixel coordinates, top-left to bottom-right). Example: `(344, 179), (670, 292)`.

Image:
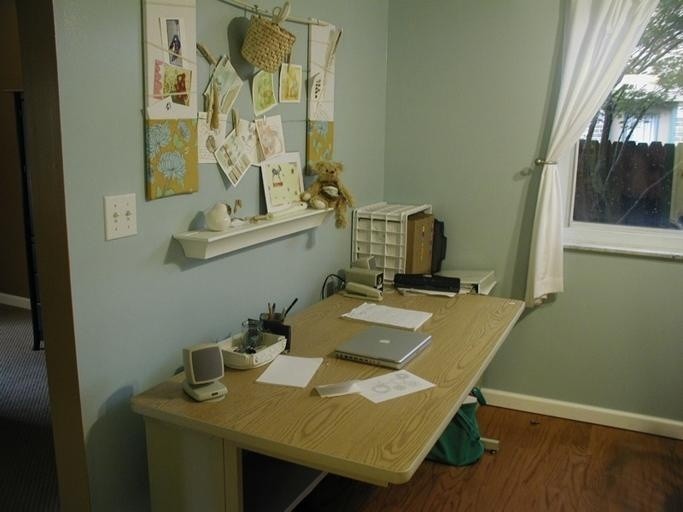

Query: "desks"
(130, 287), (526, 512)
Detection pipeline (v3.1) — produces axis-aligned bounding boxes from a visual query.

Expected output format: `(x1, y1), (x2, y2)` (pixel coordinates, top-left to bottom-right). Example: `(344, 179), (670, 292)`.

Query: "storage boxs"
(405, 214), (436, 275)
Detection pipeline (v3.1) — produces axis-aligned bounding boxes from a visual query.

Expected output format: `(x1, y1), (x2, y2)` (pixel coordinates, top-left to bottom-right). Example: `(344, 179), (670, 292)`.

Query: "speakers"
(182, 343), (228, 402)
(355, 256), (376, 270)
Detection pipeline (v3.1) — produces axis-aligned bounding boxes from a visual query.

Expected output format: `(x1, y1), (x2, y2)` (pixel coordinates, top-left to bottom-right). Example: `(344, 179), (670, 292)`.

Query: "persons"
(169, 34), (181, 64)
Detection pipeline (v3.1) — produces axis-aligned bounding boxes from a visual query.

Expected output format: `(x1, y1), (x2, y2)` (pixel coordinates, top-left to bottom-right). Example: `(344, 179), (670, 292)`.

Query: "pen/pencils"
(268, 298), (298, 322)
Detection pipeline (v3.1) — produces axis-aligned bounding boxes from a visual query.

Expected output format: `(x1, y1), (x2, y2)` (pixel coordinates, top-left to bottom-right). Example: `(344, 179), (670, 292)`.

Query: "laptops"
(336, 325), (431, 370)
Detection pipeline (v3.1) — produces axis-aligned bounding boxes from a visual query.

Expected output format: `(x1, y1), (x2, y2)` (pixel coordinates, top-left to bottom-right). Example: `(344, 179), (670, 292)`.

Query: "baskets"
(240, 14), (296, 73)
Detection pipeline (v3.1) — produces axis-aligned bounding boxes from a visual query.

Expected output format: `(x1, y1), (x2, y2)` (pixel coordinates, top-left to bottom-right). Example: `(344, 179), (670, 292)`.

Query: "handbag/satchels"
(426, 387), (486, 466)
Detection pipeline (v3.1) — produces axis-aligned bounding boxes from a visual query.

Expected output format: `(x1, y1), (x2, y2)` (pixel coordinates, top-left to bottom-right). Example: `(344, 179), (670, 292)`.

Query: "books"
(339, 302), (433, 332)
(393, 271), (461, 293)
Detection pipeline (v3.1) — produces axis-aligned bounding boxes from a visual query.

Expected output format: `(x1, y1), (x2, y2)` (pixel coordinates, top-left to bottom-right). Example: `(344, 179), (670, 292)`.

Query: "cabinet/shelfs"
(4, 88), (44, 350)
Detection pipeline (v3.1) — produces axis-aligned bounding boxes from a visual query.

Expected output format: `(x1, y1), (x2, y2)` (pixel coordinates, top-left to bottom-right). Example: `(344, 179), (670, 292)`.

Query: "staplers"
(343, 282), (384, 302)
